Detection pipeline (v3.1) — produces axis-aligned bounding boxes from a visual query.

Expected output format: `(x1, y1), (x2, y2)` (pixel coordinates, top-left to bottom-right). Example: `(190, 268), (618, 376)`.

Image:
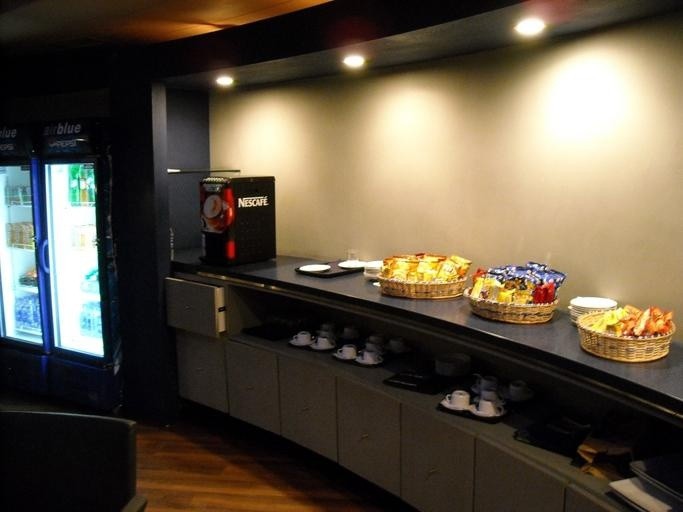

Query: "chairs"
(0, 410), (147, 512)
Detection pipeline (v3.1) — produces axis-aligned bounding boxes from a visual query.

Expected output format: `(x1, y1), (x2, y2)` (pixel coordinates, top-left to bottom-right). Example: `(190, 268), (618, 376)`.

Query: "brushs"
(197, 176), (276, 267)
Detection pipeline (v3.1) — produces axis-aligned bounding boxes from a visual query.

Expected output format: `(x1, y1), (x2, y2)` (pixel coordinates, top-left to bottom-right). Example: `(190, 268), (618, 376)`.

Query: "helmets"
(440, 399), (475, 412)
(468, 404), (507, 418)
(605, 455), (682, 512)
(298, 264), (330, 272)
(337, 261), (366, 269)
(498, 385), (536, 401)
(363, 260), (383, 280)
(470, 383), (503, 396)
(567, 296), (618, 327)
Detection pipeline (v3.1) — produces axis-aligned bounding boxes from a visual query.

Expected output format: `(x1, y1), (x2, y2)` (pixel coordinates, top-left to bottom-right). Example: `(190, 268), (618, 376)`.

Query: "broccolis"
(38, 115), (128, 413)
(0, 122), (49, 397)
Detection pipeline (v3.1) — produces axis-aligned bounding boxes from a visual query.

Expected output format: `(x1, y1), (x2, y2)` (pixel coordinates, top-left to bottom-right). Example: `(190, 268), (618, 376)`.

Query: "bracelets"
(434, 353), (471, 377)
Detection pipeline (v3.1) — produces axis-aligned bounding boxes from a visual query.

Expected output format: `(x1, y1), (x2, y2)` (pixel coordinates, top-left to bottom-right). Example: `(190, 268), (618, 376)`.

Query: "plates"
(0, 119), (128, 415)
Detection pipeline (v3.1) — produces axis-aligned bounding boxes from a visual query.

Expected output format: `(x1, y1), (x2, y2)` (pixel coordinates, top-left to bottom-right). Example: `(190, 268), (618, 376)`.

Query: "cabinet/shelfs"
(62, 162), (96, 208)
(165, 272), (683, 512)
(73, 300), (103, 337)
(0, 119), (128, 415)
(3, 178), (41, 334)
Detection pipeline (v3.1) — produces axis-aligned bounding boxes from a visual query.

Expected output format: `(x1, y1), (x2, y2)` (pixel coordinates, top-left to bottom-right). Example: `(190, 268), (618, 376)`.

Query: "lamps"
(0, 410), (147, 512)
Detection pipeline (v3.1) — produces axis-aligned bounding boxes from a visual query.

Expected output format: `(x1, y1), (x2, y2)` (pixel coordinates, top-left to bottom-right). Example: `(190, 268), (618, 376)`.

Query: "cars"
(288, 322), (384, 367)
(510, 380), (534, 401)
(480, 390), (503, 402)
(445, 390), (470, 407)
(346, 248), (359, 263)
(478, 400), (504, 415)
(476, 376), (498, 392)
